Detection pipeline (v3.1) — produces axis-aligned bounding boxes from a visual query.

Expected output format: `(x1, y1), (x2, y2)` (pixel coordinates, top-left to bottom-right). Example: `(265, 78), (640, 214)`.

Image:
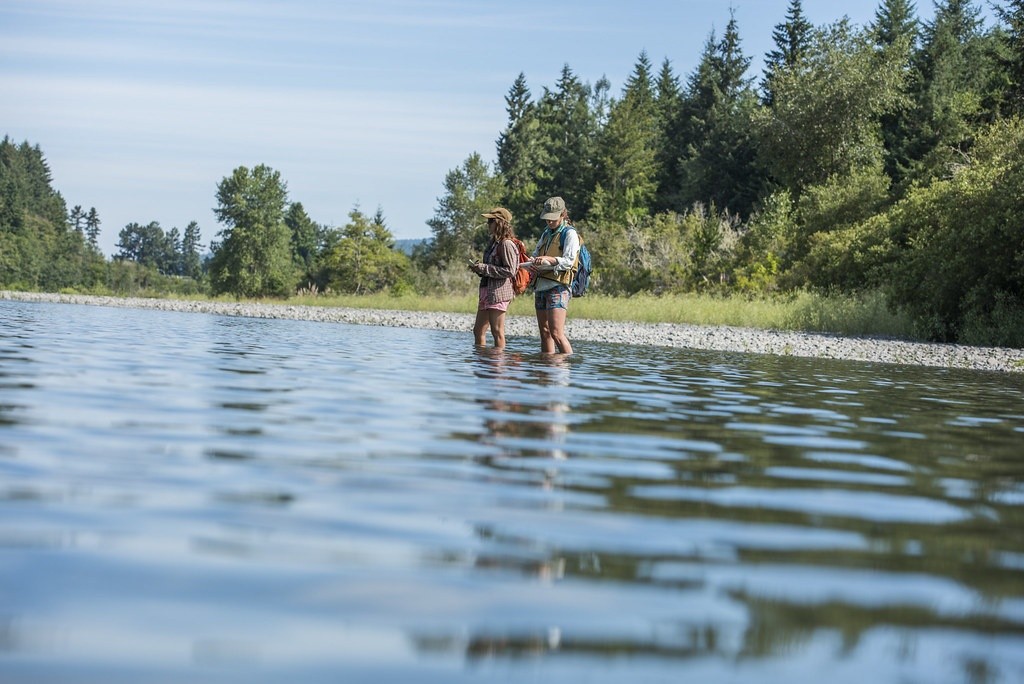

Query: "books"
(519, 260), (554, 271)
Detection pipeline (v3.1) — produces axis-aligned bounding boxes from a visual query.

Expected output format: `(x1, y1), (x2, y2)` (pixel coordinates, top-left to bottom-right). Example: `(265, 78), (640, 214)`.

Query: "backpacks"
(559, 225), (592, 297)
(502, 237), (540, 295)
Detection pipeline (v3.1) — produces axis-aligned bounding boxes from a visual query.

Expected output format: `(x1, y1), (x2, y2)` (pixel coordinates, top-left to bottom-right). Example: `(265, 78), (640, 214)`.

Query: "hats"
(540, 196), (566, 220)
(481, 208), (512, 223)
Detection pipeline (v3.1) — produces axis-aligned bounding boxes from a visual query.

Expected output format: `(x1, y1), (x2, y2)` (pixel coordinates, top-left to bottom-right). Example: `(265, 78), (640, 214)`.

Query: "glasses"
(487, 219), (495, 224)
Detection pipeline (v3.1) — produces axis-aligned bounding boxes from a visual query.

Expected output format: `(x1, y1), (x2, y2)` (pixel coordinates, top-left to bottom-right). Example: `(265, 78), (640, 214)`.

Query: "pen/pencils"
(475, 259), (479, 262)
(523, 253), (528, 258)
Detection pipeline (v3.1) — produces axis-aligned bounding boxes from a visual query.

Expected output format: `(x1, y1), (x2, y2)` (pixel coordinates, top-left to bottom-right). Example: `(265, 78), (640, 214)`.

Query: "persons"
(529, 196), (580, 355)
(473, 208), (520, 349)
